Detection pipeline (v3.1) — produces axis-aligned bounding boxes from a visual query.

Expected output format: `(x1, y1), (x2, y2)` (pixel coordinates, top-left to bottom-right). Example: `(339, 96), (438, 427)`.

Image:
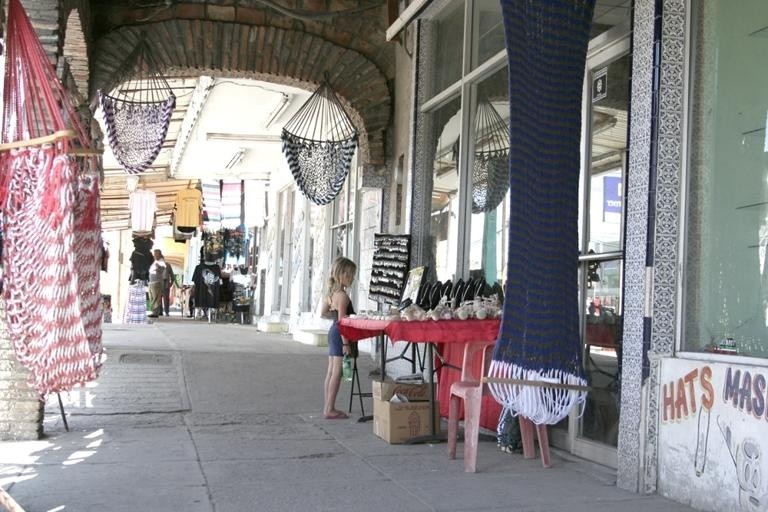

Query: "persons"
(147, 249), (195, 318)
(319, 257), (358, 418)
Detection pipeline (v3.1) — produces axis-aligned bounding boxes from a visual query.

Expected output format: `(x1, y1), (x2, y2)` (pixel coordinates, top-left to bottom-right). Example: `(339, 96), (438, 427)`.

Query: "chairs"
(447, 340), (554, 473)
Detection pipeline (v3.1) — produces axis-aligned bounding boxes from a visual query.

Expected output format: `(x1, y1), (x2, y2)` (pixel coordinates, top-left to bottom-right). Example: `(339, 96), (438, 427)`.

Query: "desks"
(334, 312), (501, 445)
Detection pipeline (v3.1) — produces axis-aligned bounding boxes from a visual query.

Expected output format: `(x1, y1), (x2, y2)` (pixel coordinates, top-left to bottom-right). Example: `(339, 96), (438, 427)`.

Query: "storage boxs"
(371, 379), (437, 402)
(372, 398), (441, 446)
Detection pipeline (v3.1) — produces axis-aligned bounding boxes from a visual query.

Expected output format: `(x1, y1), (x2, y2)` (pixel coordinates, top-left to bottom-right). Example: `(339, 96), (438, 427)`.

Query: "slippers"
(327, 410), (349, 418)
